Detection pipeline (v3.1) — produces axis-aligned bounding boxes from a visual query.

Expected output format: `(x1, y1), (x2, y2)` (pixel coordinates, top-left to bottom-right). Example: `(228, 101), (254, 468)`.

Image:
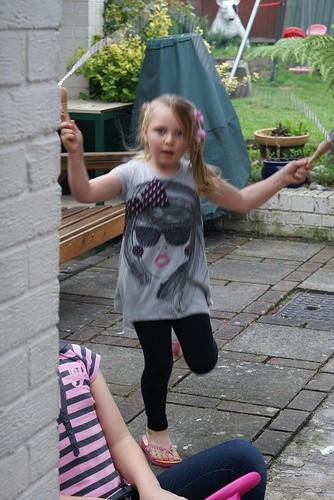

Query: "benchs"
(59, 153), (141, 266)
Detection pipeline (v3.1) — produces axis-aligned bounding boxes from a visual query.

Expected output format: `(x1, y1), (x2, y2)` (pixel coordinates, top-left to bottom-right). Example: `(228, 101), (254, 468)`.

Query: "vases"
(254, 128), (310, 161)
(262, 158), (308, 189)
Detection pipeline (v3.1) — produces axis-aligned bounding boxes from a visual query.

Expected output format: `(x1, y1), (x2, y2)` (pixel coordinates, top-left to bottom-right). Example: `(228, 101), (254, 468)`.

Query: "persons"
(60, 93), (313, 468)
(59, 339), (267, 500)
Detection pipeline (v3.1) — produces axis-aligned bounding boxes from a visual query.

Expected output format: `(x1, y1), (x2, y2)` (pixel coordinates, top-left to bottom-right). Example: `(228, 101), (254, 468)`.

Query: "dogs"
(206, 0), (251, 51)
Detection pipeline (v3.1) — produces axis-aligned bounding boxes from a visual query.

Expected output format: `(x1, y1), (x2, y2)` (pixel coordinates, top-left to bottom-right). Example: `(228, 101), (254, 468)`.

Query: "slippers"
(173, 342), (182, 362)
(141, 435), (183, 468)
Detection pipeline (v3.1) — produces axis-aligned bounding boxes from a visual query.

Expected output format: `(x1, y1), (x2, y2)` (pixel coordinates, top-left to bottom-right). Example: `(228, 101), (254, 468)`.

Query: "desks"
(59, 102), (134, 206)
